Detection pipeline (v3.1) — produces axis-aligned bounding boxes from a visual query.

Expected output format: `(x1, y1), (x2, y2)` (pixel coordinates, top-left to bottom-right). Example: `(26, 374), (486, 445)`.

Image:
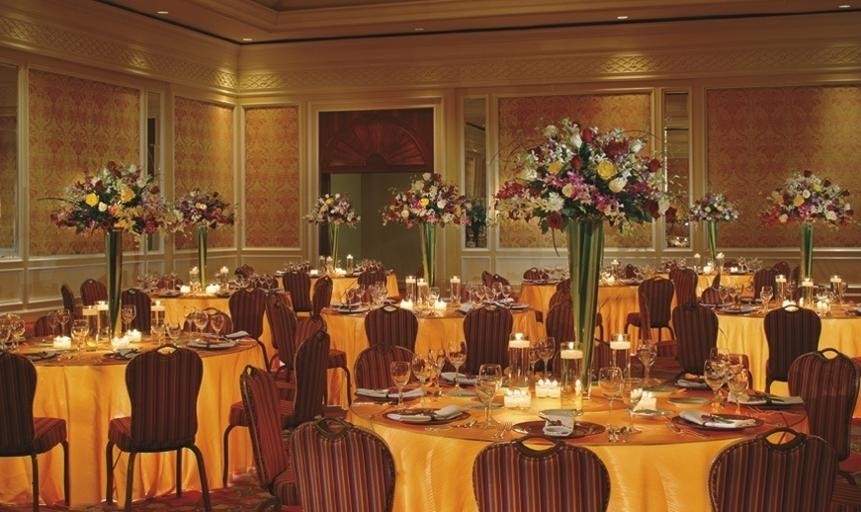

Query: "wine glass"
(703, 345), (749, 417)
(528, 338), (553, 379)
(712, 275), (847, 315)
(614, 378), (645, 435)
(635, 339), (657, 388)
(736, 254), (753, 274)
(388, 341), (468, 409)
(0, 305), (225, 353)
(631, 262), (659, 281)
(134, 266), (274, 292)
(344, 273), (515, 313)
(474, 363), (502, 431)
(598, 363), (623, 434)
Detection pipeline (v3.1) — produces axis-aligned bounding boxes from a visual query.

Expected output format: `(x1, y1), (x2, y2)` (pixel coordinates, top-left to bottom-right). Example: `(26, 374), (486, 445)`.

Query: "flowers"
(482, 115), (691, 231)
(40, 159), (174, 235)
(303, 192), (362, 225)
(685, 190), (740, 221)
(380, 171), (486, 231)
(164, 186), (236, 234)
(762, 170), (858, 226)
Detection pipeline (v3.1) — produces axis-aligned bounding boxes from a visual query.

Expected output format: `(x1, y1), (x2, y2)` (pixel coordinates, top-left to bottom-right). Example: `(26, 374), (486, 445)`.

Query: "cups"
(505, 332), (530, 408)
(559, 340), (585, 418)
(607, 335), (632, 401)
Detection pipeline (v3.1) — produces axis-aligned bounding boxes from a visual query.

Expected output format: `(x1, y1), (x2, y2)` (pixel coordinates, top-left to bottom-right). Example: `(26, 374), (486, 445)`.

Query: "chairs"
(1, 259), (860, 512)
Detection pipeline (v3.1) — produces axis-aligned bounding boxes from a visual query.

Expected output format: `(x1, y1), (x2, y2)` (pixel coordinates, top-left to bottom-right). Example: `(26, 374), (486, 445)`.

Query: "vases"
(562, 220), (603, 389)
(420, 224), (438, 288)
(101, 231), (121, 327)
(797, 222), (815, 284)
(705, 218), (719, 262)
(324, 224), (338, 268)
(193, 229), (209, 284)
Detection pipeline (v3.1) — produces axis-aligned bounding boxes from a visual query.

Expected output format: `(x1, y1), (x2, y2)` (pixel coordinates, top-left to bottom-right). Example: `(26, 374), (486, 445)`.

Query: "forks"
(498, 421), (510, 440)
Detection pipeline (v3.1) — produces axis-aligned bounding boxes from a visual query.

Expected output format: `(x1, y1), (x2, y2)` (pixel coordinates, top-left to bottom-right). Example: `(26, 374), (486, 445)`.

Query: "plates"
(511, 421), (606, 439)
(729, 394), (796, 411)
(671, 412), (761, 431)
(671, 370), (717, 390)
(382, 410), (470, 426)
(351, 388), (418, 403)
